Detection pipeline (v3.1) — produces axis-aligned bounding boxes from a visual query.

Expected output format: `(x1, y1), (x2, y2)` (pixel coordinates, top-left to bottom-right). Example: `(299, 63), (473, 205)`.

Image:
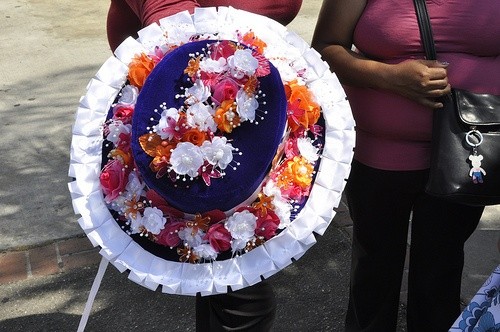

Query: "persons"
(107, 0), (303, 332)
(311, 0), (500, 332)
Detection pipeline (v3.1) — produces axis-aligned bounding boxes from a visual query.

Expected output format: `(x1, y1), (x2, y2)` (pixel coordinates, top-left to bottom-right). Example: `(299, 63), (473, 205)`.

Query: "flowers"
(98, 21), (325, 268)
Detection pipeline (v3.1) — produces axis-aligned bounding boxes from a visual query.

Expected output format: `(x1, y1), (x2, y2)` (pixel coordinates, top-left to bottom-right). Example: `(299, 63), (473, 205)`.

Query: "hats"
(67, 6), (356, 297)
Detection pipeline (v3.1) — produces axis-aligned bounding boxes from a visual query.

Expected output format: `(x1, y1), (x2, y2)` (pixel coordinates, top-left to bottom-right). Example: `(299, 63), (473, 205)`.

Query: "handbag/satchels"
(424, 86), (500, 205)
